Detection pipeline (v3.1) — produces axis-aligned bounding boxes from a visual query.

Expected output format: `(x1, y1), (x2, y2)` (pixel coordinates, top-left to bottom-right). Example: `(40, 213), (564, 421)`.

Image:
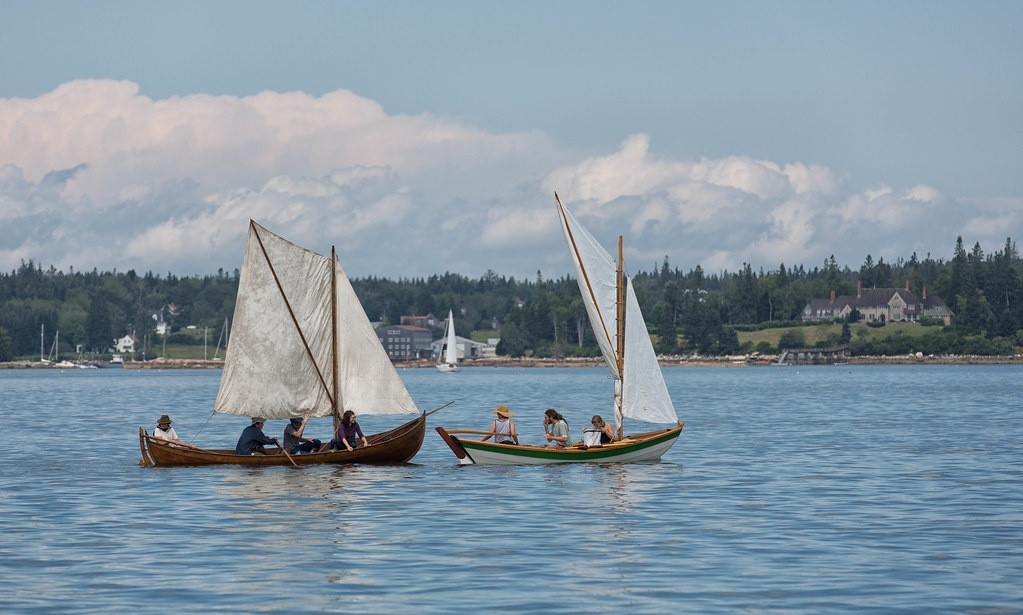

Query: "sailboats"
(41, 324), (98, 368)
(139, 218), (454, 467)
(434, 191), (686, 467)
(436, 308), (460, 372)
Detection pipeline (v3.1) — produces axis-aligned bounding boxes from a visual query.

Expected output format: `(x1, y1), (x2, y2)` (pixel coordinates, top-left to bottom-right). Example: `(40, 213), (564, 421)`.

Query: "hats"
(491, 405), (512, 417)
(156, 415), (172, 424)
(290, 416), (303, 423)
(251, 416), (267, 425)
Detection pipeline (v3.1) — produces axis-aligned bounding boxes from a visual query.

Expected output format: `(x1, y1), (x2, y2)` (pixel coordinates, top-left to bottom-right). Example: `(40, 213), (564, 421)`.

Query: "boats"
(109, 354), (124, 364)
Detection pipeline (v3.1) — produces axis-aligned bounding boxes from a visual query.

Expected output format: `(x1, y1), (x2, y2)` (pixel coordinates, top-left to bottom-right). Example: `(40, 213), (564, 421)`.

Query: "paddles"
(141, 434), (220, 455)
(275, 440), (298, 467)
(445, 429), (519, 435)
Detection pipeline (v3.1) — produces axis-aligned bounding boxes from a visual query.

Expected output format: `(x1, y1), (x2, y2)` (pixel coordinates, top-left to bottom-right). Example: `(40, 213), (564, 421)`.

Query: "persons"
(234, 415), (276, 456)
(153, 414), (186, 447)
(282, 414), (322, 456)
(479, 405), (521, 445)
(542, 408), (570, 447)
(582, 415), (616, 443)
(329, 410), (368, 452)
